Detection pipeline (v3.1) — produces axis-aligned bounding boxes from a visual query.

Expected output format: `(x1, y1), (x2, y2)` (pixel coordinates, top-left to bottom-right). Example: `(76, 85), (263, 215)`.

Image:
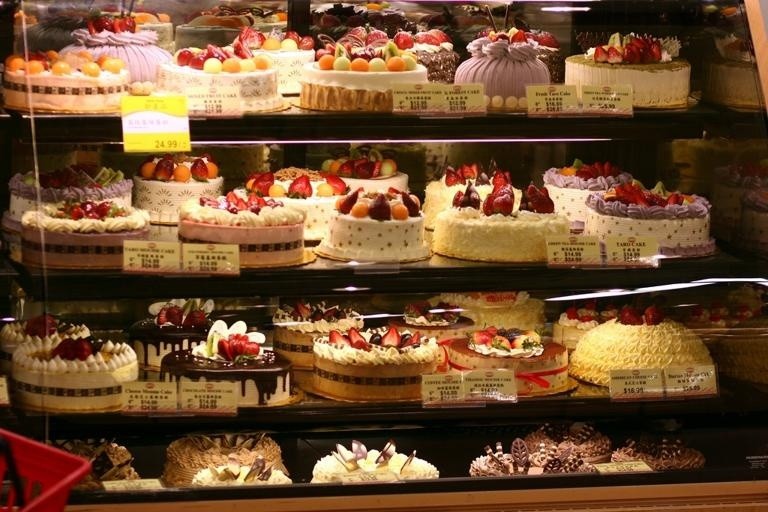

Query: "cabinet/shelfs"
(0, 1), (766, 506)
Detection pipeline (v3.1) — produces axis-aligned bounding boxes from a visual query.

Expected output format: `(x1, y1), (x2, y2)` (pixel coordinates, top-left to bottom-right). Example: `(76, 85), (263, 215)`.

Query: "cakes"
(0, 16), (768, 494)
